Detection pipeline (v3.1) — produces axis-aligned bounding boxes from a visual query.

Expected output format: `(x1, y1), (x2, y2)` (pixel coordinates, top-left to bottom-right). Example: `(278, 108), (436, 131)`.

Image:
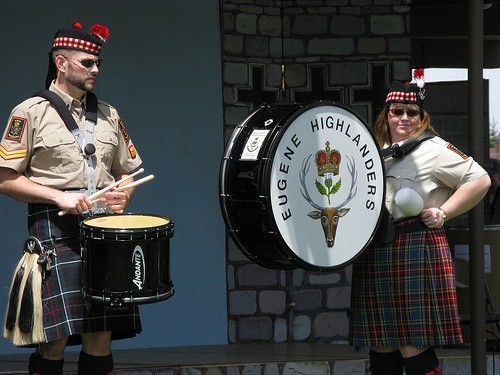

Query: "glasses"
(387, 108), (422, 117)
(63, 57), (102, 68)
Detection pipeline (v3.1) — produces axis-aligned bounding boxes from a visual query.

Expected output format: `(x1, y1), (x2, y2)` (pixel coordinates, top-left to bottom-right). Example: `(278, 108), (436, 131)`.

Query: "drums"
(75, 212), (176, 306)
(217, 100), (386, 273)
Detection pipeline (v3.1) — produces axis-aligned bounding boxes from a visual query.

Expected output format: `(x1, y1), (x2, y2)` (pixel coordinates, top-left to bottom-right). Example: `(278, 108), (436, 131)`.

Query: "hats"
(52, 22), (110, 56)
(384, 69), (426, 108)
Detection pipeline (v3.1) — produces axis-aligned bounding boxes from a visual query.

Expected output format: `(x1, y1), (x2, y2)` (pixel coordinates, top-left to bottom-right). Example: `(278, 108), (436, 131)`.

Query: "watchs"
(437, 207), (447, 220)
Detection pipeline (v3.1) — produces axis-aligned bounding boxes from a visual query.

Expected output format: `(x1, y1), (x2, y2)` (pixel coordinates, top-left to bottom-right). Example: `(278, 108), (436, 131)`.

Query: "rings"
(428, 221), (430, 226)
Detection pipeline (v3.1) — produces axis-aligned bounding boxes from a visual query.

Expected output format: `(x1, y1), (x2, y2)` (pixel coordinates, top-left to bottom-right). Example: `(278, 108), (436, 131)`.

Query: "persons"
(348, 81), (491, 374)
(0, 20), (141, 375)
(486, 158), (500, 223)
